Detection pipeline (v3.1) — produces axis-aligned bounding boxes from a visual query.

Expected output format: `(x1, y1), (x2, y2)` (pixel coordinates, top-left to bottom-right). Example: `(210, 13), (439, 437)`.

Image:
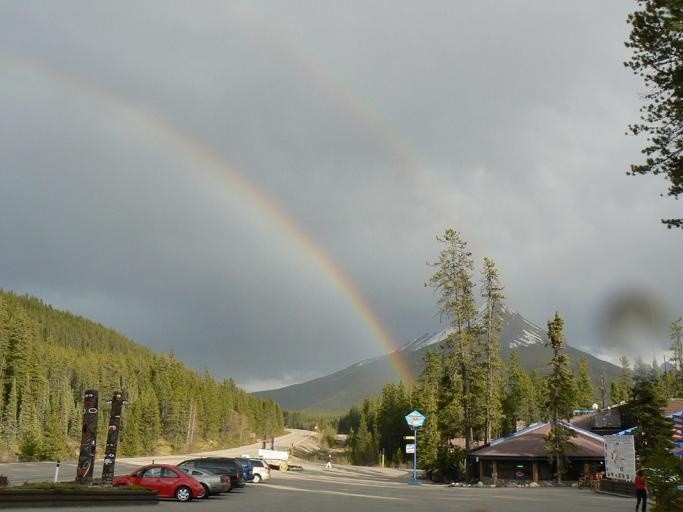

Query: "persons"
(325, 455), (332, 471)
(634, 470), (647, 512)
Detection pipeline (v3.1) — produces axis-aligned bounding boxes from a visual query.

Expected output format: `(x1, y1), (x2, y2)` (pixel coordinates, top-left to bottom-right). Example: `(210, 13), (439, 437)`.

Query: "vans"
(175, 458), (243, 493)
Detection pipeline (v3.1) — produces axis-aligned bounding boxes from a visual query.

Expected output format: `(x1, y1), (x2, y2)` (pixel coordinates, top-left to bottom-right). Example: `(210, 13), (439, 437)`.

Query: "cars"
(113, 463), (203, 503)
(173, 466), (231, 500)
(655, 481), (682, 512)
(636, 467), (680, 493)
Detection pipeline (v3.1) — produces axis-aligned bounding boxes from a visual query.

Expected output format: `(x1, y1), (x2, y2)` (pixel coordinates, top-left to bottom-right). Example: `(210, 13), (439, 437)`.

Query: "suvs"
(247, 458), (268, 484)
(234, 458), (254, 481)
